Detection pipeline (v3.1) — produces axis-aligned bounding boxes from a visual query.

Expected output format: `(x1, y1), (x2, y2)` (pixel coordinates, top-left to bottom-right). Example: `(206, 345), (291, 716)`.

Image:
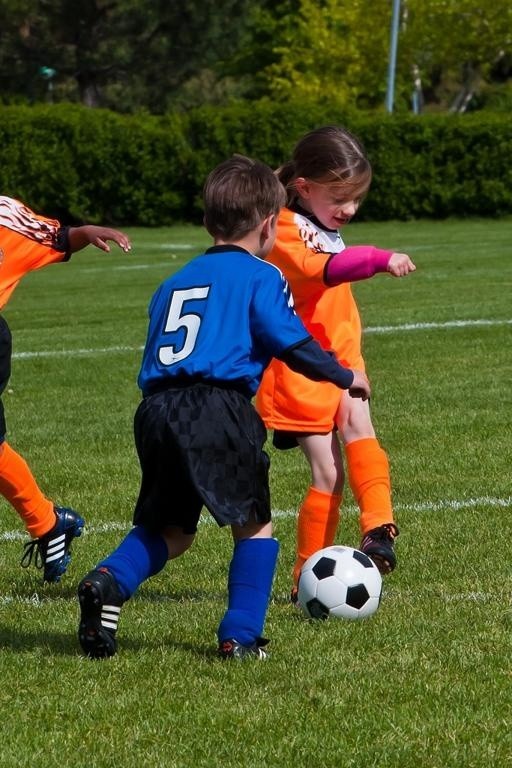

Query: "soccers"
(297, 545), (382, 620)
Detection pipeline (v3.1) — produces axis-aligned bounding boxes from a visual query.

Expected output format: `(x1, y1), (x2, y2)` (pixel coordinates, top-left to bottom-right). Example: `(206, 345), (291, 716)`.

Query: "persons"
(0, 191), (134, 581)
(76, 153), (373, 661)
(257, 126), (419, 595)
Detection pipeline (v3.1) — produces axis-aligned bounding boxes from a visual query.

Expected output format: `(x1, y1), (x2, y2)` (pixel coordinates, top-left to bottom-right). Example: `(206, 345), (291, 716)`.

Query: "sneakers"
(357, 523), (399, 574)
(78, 567), (124, 660)
(38, 507), (85, 585)
(218, 637), (271, 666)
